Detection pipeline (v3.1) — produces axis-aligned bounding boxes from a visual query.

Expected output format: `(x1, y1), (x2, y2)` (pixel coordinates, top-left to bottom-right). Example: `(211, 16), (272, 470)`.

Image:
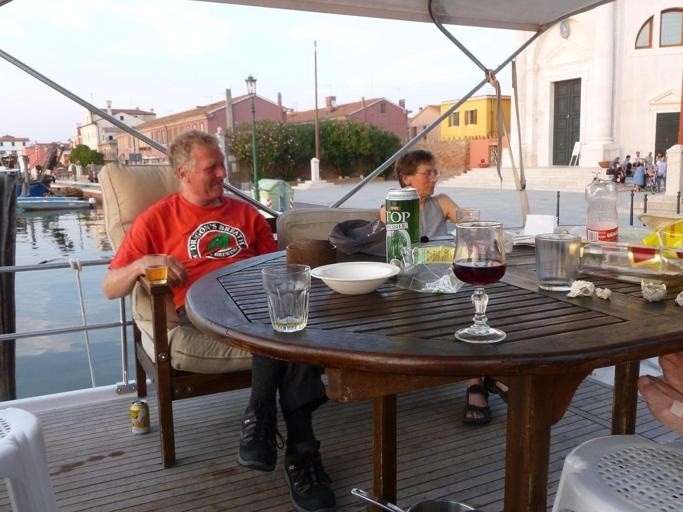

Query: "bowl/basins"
(310, 259), (401, 299)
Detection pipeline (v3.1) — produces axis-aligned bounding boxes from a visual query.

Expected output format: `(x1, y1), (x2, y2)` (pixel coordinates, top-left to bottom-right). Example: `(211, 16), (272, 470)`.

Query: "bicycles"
(648, 167), (658, 195)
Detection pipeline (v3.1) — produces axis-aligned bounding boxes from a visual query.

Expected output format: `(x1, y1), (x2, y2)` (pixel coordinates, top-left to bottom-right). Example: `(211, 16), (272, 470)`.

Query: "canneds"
(130, 399), (151, 435)
(384, 187), (421, 276)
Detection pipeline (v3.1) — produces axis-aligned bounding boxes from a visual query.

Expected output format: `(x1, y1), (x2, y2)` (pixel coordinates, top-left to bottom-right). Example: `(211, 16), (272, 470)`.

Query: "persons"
(610, 148), (666, 194)
(101, 127), (340, 511)
(635, 352), (682, 439)
(86, 168), (99, 183)
(379, 146), (509, 426)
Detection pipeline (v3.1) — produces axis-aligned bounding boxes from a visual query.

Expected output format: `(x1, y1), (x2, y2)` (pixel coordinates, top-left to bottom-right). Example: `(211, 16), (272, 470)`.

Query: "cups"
(143, 253), (167, 285)
(532, 233), (582, 294)
(455, 205), (480, 223)
(261, 263), (313, 335)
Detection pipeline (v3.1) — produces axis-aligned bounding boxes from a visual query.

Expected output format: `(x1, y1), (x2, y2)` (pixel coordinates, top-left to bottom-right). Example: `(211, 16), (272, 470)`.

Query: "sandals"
(483, 375), (509, 403)
(461, 384), (490, 424)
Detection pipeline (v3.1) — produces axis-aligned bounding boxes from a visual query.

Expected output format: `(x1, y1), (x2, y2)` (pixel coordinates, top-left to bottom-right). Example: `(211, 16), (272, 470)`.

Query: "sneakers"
(237, 406), (283, 471)
(282, 440), (336, 511)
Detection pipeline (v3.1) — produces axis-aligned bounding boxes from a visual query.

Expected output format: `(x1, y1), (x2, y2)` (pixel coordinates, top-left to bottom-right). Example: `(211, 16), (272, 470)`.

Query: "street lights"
(245, 75), (260, 203)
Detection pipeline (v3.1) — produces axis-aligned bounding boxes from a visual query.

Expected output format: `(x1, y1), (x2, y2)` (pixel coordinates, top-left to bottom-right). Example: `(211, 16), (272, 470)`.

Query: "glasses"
(416, 171), (440, 177)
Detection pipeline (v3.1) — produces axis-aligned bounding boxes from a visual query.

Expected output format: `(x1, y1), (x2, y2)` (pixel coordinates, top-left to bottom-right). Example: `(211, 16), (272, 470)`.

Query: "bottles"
(585, 180), (619, 241)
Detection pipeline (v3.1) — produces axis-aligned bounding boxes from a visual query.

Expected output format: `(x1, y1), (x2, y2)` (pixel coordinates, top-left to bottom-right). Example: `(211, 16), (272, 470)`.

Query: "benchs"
(97, 161), (381, 472)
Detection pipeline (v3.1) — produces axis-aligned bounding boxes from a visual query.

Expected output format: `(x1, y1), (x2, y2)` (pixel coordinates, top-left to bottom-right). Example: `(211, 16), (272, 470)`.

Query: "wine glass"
(452, 221), (509, 343)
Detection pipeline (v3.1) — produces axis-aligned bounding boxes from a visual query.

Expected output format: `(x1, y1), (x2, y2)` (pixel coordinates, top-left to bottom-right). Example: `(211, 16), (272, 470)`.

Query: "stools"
(0, 405), (57, 512)
(549, 430), (683, 512)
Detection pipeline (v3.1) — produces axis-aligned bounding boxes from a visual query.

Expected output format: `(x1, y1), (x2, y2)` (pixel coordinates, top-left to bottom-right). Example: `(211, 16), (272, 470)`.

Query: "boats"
(15, 196), (95, 210)
